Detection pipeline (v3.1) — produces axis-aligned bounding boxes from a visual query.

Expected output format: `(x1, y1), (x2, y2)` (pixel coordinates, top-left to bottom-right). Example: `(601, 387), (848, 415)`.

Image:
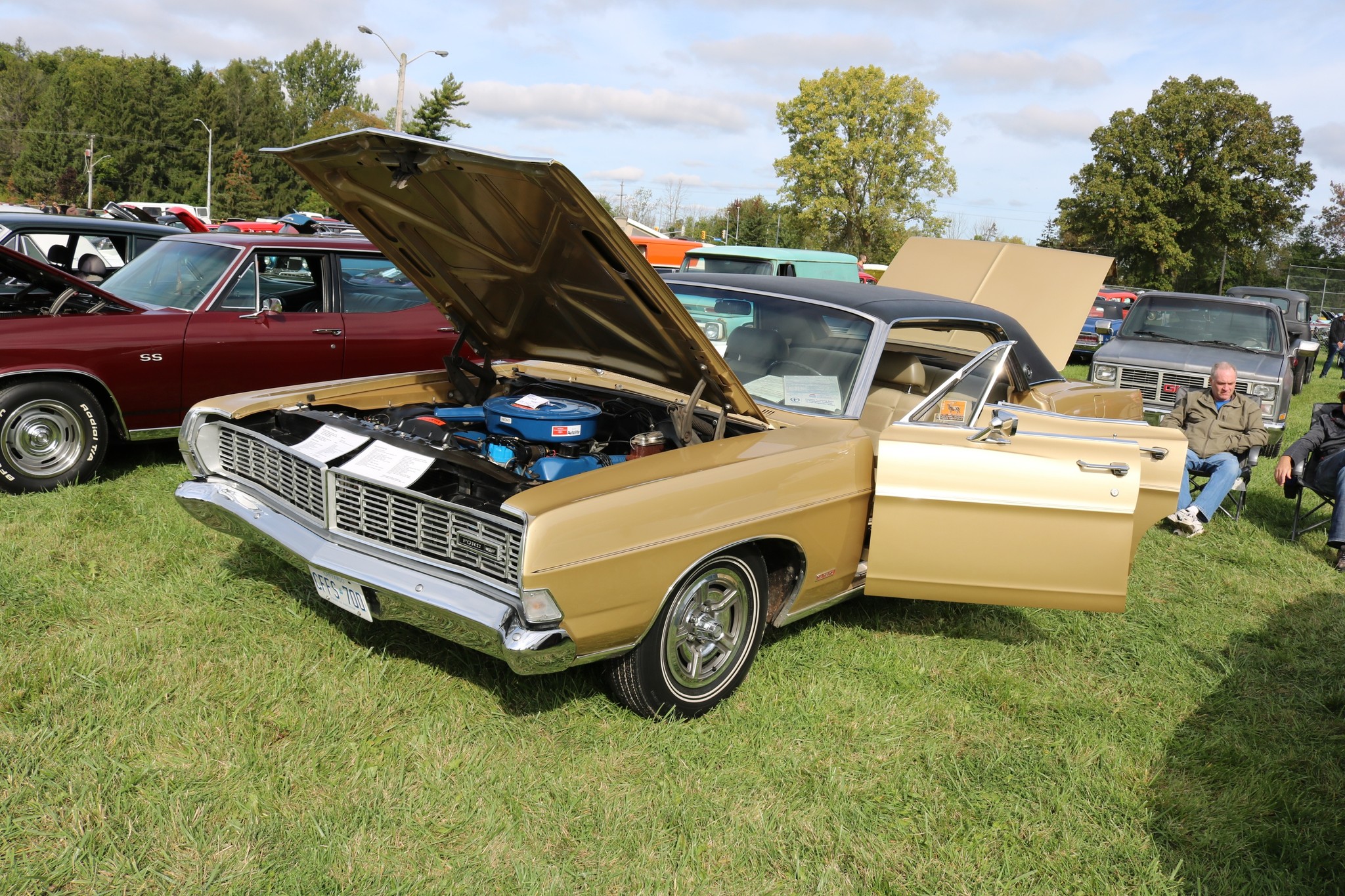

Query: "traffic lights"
(722, 230), (726, 239)
(702, 231), (705, 240)
(655, 227), (658, 232)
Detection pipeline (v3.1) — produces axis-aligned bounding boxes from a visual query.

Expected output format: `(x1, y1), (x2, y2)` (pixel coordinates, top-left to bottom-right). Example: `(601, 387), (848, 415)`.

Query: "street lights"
(358, 25), (449, 132)
(714, 211), (730, 245)
(193, 119), (212, 217)
(88, 155), (111, 208)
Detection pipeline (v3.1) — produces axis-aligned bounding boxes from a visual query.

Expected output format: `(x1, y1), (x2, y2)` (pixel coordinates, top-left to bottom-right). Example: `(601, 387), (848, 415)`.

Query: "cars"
(0, 202), (524, 495)
(172, 127), (1191, 724)
(1072, 286), (1320, 459)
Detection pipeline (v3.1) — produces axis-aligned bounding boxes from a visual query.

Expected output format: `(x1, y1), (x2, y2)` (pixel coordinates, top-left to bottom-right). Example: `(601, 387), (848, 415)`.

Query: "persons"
(1274, 391), (1345, 573)
(1100, 285), (1106, 289)
(1158, 361), (1269, 539)
(857, 254), (866, 273)
(21, 201), (96, 217)
(1319, 311), (1345, 380)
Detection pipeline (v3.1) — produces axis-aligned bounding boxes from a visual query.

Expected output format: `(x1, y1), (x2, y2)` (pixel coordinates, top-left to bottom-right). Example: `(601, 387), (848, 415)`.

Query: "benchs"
(343, 292), (425, 312)
(771, 347), (1010, 428)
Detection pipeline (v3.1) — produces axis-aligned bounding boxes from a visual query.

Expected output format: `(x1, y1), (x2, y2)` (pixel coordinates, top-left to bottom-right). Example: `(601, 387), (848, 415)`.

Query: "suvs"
(664, 245), (862, 360)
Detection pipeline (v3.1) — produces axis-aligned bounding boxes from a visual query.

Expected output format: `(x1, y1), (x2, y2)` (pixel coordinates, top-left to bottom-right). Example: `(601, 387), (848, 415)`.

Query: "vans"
(628, 235), (717, 270)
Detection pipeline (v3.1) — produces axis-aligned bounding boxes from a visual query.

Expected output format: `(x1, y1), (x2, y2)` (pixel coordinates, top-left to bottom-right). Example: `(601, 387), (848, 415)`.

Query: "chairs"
(242, 293), (285, 312)
(47, 244), (71, 273)
(1285, 402), (1341, 543)
(723, 327), (790, 385)
(75, 254), (105, 281)
(1174, 387), (1263, 523)
(859, 352), (940, 485)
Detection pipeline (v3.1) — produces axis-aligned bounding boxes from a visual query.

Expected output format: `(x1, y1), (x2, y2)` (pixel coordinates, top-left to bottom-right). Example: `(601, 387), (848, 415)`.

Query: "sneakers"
(1336, 545), (1345, 572)
(1174, 517), (1205, 538)
(1167, 508), (1196, 533)
